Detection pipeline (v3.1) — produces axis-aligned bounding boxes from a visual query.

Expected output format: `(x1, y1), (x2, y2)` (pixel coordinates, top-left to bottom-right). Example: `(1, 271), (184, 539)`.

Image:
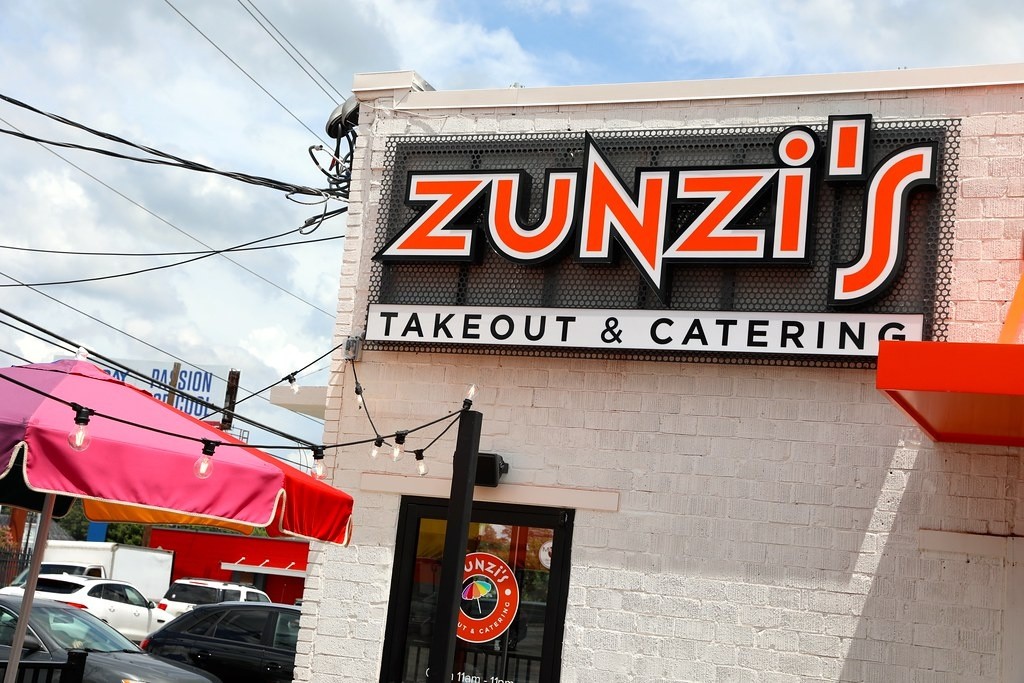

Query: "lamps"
(454, 451), (509, 488)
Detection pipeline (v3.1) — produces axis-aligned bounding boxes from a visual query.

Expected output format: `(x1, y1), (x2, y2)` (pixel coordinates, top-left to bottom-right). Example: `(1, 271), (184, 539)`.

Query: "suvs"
(155, 578), (272, 617)
(139, 604), (302, 683)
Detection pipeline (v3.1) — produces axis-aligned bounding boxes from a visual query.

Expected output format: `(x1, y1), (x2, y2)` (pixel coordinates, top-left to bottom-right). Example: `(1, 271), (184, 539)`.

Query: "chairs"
(2, 619), (19, 644)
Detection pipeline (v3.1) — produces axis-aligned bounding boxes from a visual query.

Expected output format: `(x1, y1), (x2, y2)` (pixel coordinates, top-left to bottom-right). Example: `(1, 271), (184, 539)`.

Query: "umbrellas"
(0, 345), (354, 683)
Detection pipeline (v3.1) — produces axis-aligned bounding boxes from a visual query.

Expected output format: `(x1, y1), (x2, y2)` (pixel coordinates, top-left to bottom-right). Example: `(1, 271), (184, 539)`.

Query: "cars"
(0, 593), (221, 683)
(0, 575), (177, 641)
(410, 593), (530, 651)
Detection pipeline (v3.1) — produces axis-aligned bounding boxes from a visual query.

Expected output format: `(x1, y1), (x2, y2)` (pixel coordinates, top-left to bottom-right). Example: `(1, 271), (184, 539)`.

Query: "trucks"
(11, 541), (175, 604)
